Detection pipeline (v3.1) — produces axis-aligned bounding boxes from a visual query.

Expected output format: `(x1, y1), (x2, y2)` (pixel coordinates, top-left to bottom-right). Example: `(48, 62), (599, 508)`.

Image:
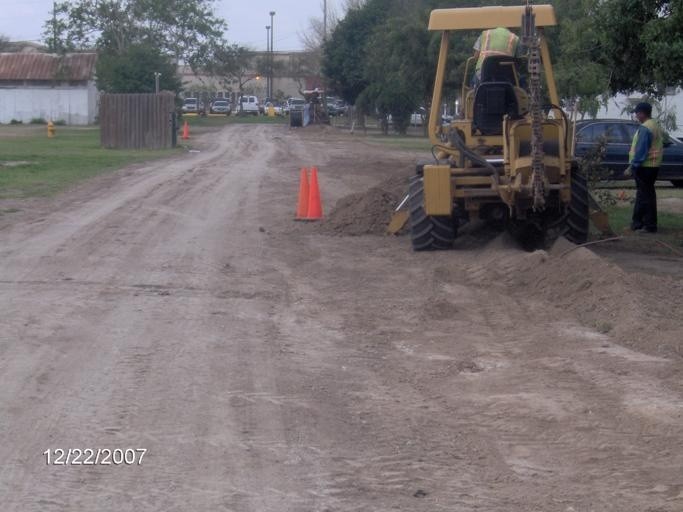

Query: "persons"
(624, 101), (663, 233)
(469, 27), (522, 87)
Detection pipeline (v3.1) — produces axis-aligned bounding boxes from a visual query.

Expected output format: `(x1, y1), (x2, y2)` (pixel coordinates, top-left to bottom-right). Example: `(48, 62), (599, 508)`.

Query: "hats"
(630, 103), (652, 113)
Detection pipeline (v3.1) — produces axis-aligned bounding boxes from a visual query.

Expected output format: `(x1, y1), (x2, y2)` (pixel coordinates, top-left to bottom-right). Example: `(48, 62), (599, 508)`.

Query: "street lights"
(268, 11), (275, 95)
(264, 25), (270, 97)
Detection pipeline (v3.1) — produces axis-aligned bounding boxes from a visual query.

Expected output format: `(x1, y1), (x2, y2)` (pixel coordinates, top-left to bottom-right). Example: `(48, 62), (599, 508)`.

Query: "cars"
(182, 94), (348, 118)
(384, 104), (451, 127)
(567, 113), (682, 188)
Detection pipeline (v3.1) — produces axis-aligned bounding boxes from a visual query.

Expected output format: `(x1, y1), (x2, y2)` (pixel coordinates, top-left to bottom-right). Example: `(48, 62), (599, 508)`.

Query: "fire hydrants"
(45, 118), (55, 138)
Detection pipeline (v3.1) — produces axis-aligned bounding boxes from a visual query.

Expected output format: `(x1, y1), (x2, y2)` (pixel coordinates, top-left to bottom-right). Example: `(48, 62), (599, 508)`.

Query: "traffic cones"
(179, 120), (190, 140)
(291, 164), (309, 221)
(307, 166), (327, 220)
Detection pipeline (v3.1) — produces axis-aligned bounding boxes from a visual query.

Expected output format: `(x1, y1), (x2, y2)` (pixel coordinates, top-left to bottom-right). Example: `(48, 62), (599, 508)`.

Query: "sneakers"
(635, 227), (648, 233)
(622, 224), (634, 233)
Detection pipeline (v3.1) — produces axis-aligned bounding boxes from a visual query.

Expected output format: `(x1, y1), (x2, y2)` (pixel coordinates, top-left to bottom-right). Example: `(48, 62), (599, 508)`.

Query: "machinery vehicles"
(383, 0), (592, 253)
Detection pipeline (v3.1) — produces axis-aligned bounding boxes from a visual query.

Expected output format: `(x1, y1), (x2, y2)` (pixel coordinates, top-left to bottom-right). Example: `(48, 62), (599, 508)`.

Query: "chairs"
(478, 53), (520, 86)
